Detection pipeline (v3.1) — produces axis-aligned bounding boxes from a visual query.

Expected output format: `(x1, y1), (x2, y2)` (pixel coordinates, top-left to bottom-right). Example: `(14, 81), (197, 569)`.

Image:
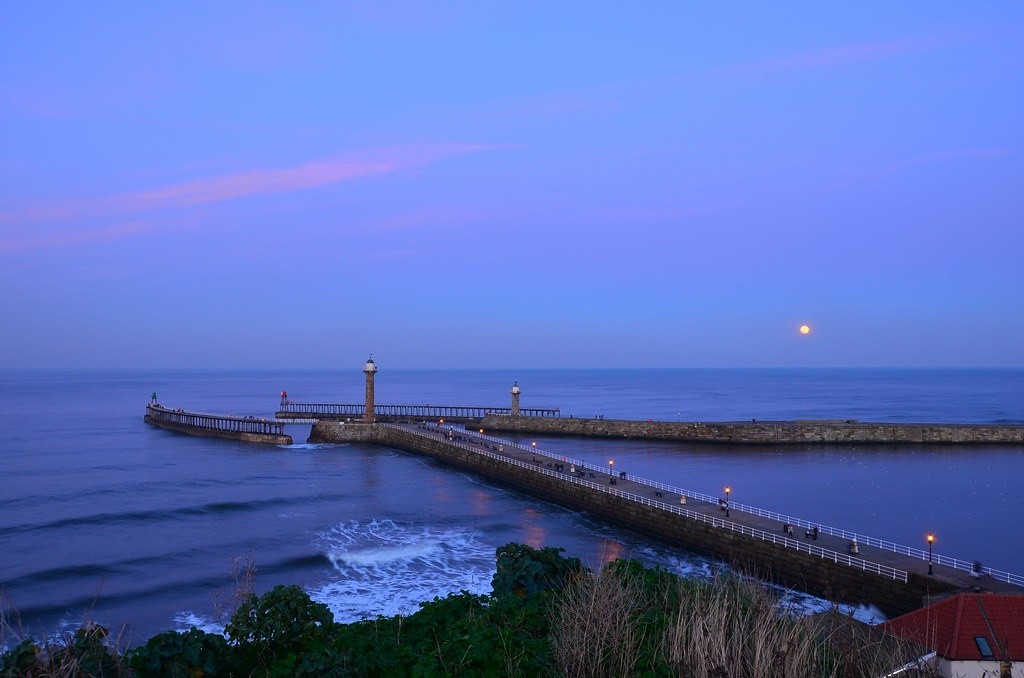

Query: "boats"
(144, 392), (294, 446)
(274, 391), (560, 423)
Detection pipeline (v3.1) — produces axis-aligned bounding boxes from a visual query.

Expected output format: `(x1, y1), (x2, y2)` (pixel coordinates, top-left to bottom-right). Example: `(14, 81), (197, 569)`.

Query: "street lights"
(440, 419), (443, 434)
(532, 441), (536, 461)
(725, 486), (730, 517)
(609, 459), (613, 483)
(480, 429), (483, 446)
(927, 533), (935, 575)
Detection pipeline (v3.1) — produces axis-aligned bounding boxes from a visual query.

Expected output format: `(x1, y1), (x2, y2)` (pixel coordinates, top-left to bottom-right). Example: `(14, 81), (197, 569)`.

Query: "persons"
(596, 414), (604, 419)
(570, 413), (572, 418)
(788, 524), (793, 538)
